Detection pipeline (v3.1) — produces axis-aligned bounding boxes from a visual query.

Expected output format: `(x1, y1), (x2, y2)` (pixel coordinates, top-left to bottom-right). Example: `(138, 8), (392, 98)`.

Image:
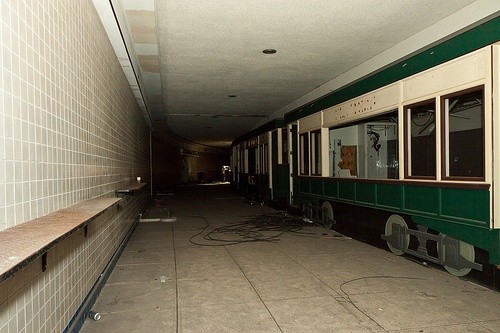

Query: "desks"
(0, 195), (124, 285)
(114, 182), (148, 194)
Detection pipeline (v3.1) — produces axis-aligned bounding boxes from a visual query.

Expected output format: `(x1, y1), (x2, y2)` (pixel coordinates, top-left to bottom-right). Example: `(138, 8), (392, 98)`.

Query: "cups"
(137, 176), (141, 181)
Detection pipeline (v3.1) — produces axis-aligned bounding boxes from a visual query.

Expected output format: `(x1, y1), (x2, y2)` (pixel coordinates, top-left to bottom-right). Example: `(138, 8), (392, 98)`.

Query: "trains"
(230, 14), (500, 278)
